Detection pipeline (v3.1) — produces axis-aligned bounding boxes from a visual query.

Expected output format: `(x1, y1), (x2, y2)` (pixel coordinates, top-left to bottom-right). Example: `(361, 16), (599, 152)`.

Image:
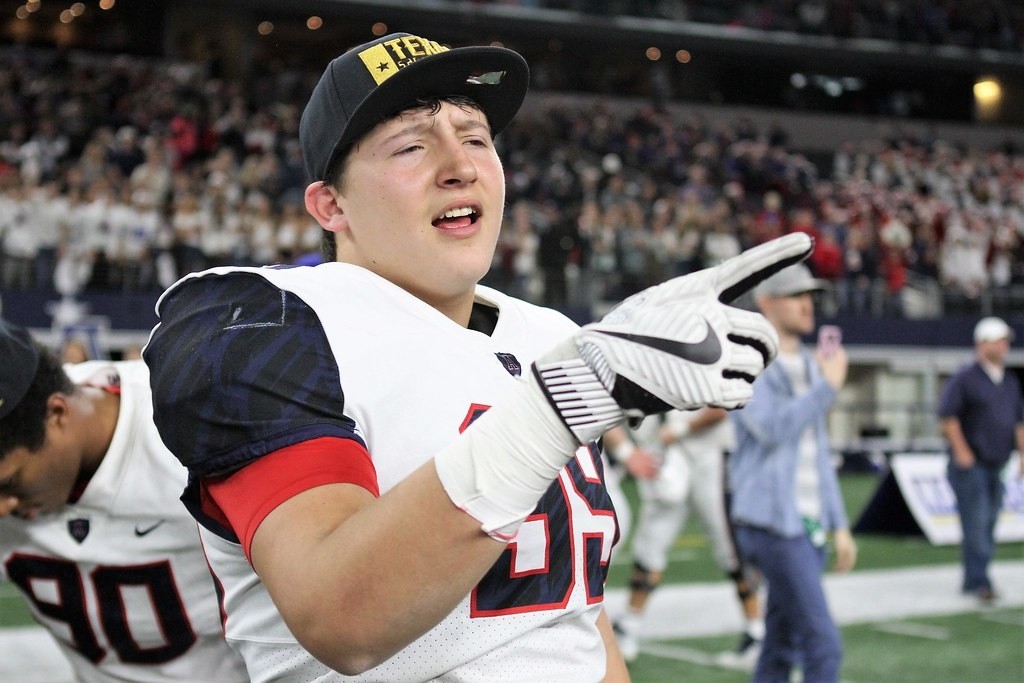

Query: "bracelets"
(674, 420), (691, 436)
(612, 441), (634, 460)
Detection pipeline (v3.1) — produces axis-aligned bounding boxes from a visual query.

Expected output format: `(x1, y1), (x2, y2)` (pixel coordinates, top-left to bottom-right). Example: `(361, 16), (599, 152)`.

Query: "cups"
(819, 325), (841, 360)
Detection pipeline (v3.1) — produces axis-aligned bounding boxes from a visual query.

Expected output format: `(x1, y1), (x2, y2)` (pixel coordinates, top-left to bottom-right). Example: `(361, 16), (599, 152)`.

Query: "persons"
(141, 33), (815, 683)
(0, 51), (1024, 319)
(732, 265), (855, 683)
(0, 318), (252, 683)
(936, 317), (1024, 601)
(602, 408), (764, 659)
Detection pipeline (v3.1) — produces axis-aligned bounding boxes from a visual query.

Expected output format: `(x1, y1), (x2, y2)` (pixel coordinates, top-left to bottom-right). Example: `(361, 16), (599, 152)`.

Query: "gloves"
(531, 230), (817, 447)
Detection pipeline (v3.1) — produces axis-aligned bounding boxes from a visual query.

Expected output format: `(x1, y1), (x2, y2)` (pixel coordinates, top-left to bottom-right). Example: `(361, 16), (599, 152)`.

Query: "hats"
(972, 317), (1014, 344)
(299, 32), (531, 186)
(756, 262), (827, 301)
(1, 313), (38, 416)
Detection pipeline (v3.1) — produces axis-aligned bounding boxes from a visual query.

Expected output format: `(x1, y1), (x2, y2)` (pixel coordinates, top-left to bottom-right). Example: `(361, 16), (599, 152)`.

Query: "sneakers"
(715, 619), (767, 670)
(612, 606), (641, 661)
